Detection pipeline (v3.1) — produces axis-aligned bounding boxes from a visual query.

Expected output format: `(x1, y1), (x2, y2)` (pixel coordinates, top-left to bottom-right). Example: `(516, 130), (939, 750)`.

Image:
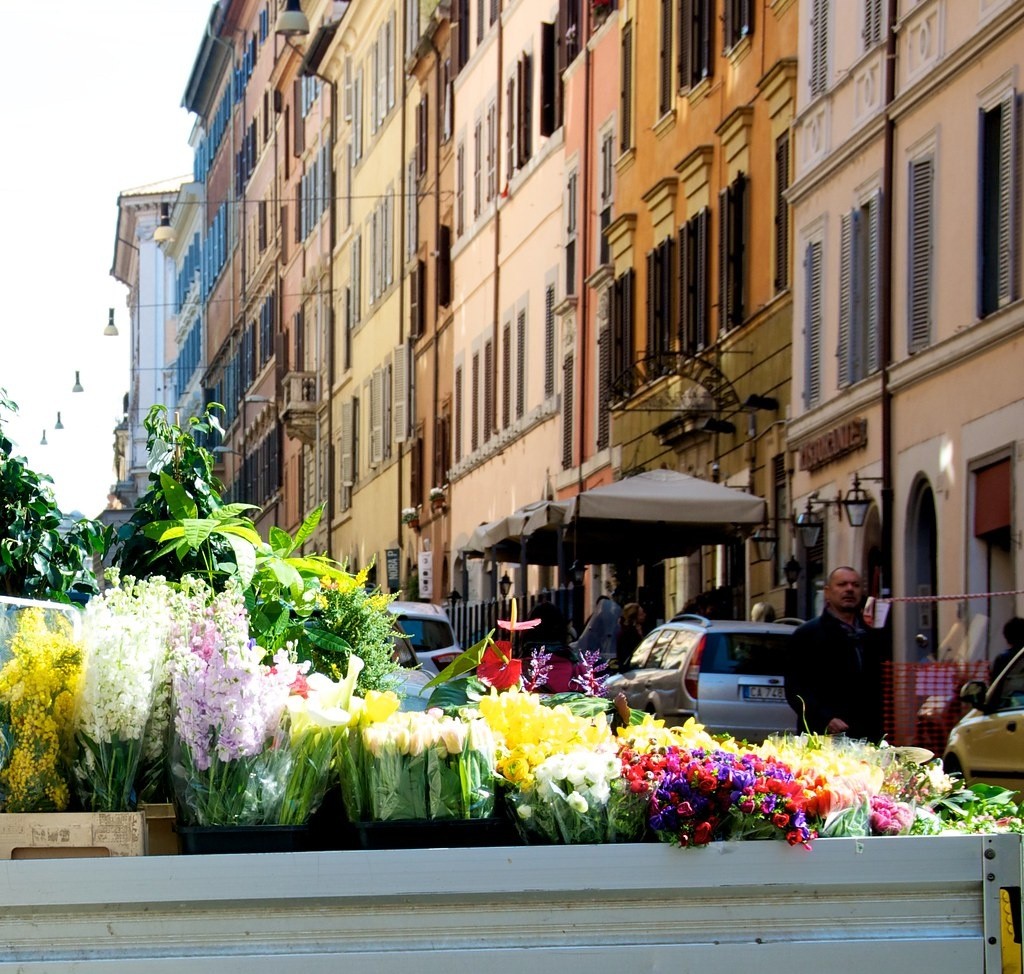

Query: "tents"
(461, 468), (765, 601)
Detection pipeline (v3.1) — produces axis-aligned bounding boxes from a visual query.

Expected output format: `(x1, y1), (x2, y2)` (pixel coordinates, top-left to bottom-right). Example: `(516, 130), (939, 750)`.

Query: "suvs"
(598, 614), (807, 746)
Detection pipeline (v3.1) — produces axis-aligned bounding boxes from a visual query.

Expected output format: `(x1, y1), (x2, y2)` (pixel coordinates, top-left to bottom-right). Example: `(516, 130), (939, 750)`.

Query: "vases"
(407, 519), (420, 528)
(138, 804), (180, 854)
(431, 498), (447, 513)
(0, 805), (147, 856)
(353, 817), (510, 849)
(167, 819), (311, 853)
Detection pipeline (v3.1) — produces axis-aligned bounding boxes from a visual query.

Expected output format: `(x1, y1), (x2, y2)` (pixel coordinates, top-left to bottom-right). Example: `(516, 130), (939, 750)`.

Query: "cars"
(388, 601), (465, 678)
(941, 647), (1024, 806)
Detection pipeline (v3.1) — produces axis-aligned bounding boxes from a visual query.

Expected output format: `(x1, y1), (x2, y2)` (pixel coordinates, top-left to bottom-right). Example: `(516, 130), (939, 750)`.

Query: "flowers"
(399, 507), (419, 524)
(0, 567), (1024, 854)
(428, 487), (447, 501)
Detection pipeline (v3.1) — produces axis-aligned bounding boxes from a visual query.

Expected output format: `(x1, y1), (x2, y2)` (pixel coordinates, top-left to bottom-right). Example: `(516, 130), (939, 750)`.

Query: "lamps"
(275, 0), (310, 37)
(152, 202), (177, 242)
(212, 446), (243, 456)
(54, 412), (64, 430)
(103, 308), (120, 336)
(701, 416), (738, 436)
(744, 393), (780, 412)
(243, 394), (275, 407)
(842, 472), (885, 527)
(498, 570), (513, 599)
(749, 508), (797, 561)
(794, 490), (842, 548)
(40, 430), (48, 445)
(71, 370), (84, 393)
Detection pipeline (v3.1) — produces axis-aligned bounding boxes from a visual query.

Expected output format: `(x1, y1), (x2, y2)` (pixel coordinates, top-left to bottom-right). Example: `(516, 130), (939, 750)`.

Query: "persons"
(520, 601), (597, 695)
(569, 595), (648, 670)
(752, 602), (776, 623)
(678, 586), (743, 620)
(989, 617), (1024, 697)
(966, 684), (984, 703)
(784, 566), (886, 744)
